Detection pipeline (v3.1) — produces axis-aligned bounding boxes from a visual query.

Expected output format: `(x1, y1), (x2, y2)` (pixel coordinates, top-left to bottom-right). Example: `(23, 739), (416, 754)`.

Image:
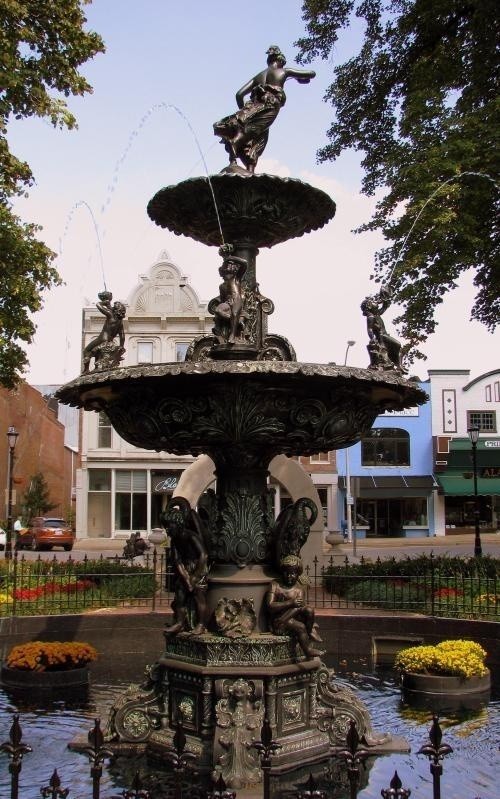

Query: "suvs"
(18, 516), (74, 552)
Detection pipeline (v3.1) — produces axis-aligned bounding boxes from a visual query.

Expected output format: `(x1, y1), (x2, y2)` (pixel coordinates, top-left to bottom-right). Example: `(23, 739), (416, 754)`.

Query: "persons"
(213, 45), (316, 175)
(159, 510), (209, 636)
(265, 555), (327, 657)
(361, 288), (409, 375)
(211, 255), (247, 345)
(81, 300), (126, 374)
(14, 516), (22, 551)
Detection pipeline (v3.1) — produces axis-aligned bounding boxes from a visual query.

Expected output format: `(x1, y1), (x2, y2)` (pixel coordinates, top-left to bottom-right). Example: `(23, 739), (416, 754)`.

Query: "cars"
(0, 527), (6, 550)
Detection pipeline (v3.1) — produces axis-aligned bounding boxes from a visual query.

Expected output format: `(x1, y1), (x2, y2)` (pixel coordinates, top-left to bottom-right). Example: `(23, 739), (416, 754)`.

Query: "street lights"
(4, 426), (20, 561)
(10, 519), (22, 616)
(466, 426), (484, 558)
(343, 339), (357, 543)
